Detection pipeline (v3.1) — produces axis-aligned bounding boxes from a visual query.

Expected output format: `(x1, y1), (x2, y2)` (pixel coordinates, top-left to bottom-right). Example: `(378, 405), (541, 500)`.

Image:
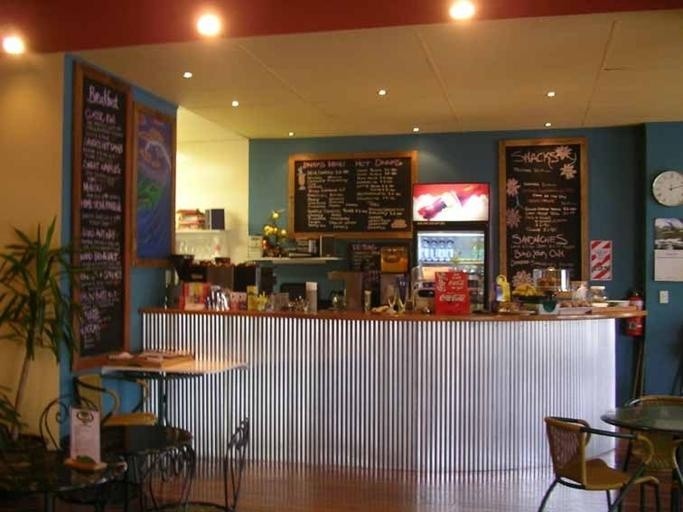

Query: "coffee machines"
(326, 270), (363, 311)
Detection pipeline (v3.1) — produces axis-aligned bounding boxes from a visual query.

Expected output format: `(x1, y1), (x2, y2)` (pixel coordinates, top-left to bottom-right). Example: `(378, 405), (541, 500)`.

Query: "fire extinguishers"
(624, 288), (644, 337)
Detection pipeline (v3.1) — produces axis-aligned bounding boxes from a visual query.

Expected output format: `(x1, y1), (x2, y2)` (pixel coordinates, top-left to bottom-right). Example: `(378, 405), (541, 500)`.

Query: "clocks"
(650, 169), (682, 209)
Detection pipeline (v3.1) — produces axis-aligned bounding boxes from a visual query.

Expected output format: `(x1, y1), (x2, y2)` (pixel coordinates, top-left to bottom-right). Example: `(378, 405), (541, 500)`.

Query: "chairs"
(72, 370), (157, 428)
(537, 417), (664, 512)
(151, 418), (250, 511)
(38, 396), (145, 510)
(624, 393), (683, 511)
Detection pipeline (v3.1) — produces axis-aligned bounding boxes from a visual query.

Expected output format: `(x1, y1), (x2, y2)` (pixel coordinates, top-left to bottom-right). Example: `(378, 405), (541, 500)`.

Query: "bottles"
(384, 247), (406, 263)
(462, 264), (484, 279)
(420, 235), (455, 262)
(395, 273), (405, 301)
(205, 285), (229, 312)
(418, 182), (480, 218)
(469, 235), (485, 261)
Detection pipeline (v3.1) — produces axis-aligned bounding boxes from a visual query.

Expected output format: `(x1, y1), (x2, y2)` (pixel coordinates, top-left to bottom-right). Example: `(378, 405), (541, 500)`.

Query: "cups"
(245, 285), (258, 310)
(532, 268), (542, 282)
(590, 286), (607, 302)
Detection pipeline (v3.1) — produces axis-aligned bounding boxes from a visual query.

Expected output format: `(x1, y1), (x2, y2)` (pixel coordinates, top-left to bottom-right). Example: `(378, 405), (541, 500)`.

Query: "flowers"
(261, 209), (287, 249)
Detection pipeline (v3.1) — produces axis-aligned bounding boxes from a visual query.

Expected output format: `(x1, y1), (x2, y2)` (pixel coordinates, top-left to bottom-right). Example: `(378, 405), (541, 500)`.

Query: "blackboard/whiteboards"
(499, 137), (590, 291)
(286, 150), (419, 241)
(70, 61), (133, 373)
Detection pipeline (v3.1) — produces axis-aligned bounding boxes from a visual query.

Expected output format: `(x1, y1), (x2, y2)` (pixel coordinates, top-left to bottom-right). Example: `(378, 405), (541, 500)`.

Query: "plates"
(558, 306), (594, 315)
(608, 300), (631, 307)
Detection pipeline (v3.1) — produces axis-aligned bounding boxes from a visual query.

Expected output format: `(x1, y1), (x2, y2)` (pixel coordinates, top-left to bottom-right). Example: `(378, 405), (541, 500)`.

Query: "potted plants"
(1, 208), (100, 492)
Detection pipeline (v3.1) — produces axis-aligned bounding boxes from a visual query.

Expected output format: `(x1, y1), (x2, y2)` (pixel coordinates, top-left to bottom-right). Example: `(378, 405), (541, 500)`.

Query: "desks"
(92, 354), (247, 511)
(593, 405), (680, 512)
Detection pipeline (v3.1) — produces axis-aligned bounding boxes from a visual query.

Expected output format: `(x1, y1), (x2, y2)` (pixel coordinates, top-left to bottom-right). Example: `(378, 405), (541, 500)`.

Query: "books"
(103, 350), (194, 370)
(435, 271), (471, 317)
(67, 406), (100, 460)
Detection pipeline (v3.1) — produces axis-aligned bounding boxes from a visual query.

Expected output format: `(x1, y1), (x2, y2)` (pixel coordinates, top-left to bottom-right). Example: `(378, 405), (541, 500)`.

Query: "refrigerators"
(410, 181), (494, 313)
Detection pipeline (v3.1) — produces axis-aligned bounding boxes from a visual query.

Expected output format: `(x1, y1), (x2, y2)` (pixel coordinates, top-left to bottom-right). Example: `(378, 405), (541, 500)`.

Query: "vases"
(265, 245), (282, 258)
